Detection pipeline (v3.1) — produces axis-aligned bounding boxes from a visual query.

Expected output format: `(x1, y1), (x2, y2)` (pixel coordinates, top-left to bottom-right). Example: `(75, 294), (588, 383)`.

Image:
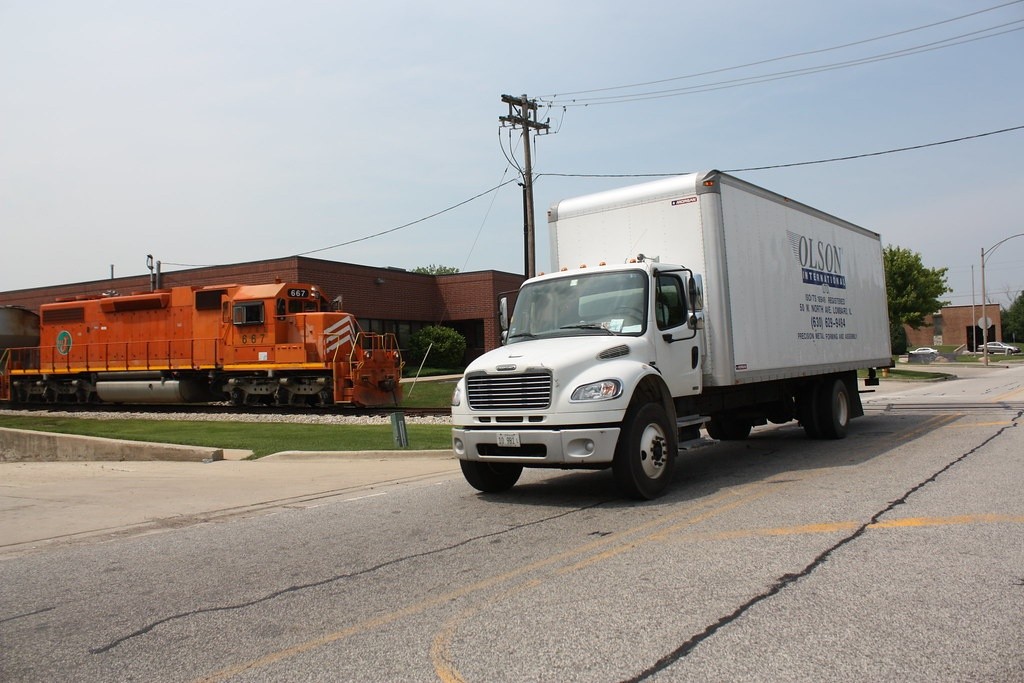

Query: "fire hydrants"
(881, 367), (889, 378)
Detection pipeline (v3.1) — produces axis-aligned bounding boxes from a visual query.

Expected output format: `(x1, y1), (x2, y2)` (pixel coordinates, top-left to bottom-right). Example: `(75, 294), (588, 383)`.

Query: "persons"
(631, 287), (669, 326)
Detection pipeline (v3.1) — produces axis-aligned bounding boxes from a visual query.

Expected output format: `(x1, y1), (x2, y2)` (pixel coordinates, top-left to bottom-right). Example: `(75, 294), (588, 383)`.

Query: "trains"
(0, 275), (406, 412)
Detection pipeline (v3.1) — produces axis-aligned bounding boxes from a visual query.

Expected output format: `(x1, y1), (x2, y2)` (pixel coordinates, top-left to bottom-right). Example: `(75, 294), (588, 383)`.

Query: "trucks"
(448, 168), (897, 501)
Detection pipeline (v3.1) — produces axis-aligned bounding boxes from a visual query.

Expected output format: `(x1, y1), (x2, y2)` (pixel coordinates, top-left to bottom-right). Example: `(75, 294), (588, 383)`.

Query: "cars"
(976, 341), (1022, 355)
(907, 346), (940, 356)
